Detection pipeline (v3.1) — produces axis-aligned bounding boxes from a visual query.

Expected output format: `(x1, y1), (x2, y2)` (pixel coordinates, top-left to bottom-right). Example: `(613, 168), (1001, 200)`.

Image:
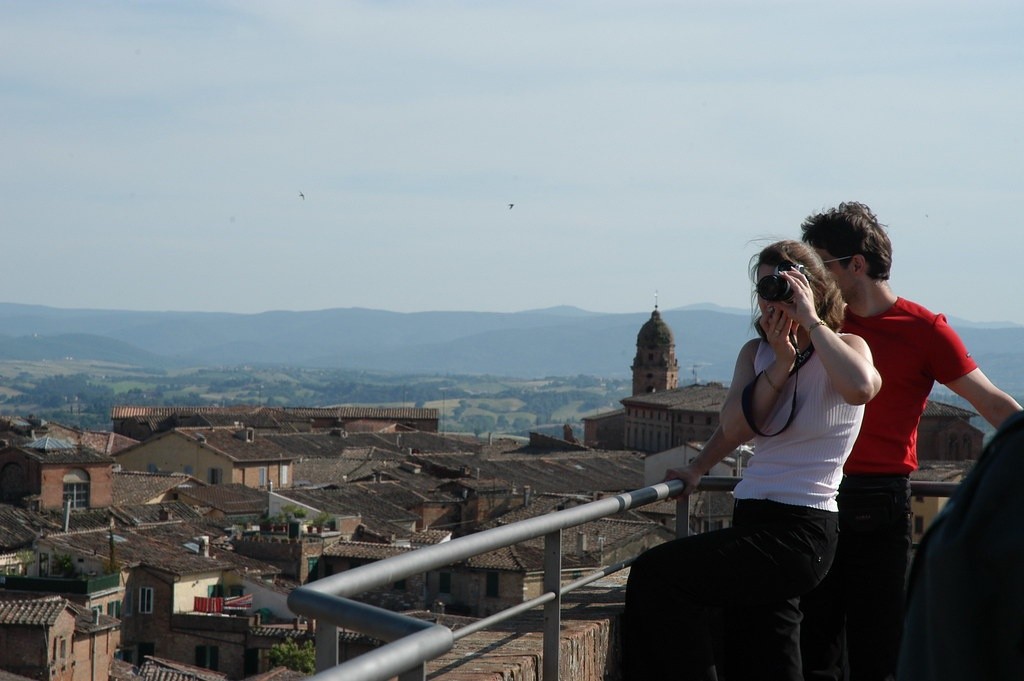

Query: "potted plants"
(307, 508), (335, 533)
(258, 503), (309, 539)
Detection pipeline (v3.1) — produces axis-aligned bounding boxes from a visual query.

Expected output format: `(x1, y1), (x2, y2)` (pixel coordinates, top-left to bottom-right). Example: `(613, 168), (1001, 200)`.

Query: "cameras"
(757, 258), (795, 302)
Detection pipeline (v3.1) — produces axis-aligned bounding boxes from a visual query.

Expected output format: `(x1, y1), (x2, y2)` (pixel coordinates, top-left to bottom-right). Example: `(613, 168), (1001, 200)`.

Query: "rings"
(805, 281), (808, 284)
(775, 329), (781, 334)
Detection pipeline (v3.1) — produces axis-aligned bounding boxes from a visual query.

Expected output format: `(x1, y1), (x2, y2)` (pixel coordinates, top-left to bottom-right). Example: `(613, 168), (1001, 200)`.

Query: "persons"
(800, 201), (1024, 680)
(618, 240), (882, 680)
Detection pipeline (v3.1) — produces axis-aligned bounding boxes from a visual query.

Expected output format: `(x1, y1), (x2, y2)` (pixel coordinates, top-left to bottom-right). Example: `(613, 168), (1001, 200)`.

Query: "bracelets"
(808, 321), (827, 336)
(762, 369), (783, 394)
